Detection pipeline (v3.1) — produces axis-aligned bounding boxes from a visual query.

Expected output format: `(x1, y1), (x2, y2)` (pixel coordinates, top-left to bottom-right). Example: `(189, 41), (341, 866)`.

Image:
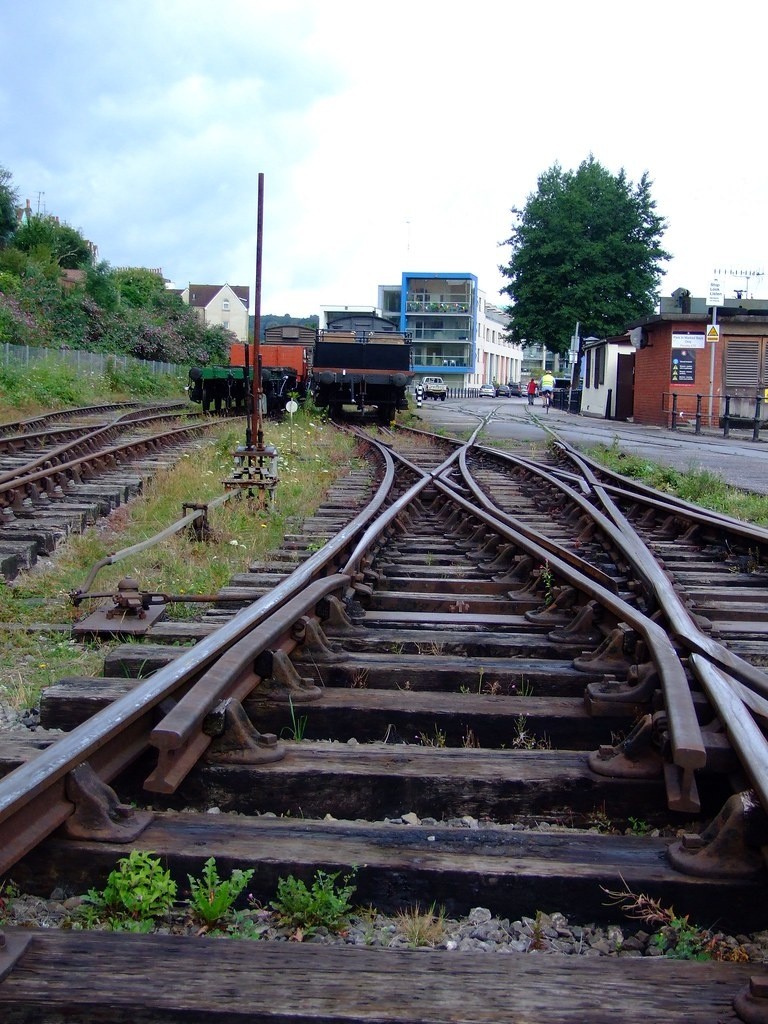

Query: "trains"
(311, 311), (414, 421)
(186, 325), (319, 412)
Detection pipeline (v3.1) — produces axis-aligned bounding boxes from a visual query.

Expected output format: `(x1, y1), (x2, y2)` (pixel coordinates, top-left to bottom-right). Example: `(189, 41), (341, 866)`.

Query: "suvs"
(508, 383), (522, 397)
(496, 386), (511, 398)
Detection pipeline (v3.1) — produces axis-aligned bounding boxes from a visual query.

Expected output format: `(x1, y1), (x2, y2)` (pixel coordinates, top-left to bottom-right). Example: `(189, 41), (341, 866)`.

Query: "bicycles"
(544, 390), (550, 413)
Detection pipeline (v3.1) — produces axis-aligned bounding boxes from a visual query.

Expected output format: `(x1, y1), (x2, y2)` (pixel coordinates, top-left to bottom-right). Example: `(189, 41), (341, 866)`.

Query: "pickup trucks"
(415, 377), (449, 401)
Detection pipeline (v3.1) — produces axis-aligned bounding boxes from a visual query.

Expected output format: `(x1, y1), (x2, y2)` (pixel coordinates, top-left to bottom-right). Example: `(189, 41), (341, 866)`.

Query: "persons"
(526, 378), (537, 405)
(496, 387), (500, 397)
(539, 370), (556, 408)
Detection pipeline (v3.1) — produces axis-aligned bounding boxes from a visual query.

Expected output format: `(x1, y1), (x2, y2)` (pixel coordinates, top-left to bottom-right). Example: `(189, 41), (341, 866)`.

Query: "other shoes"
(549, 400), (553, 404)
(531, 402), (533, 405)
(529, 403), (530, 405)
(542, 406), (545, 408)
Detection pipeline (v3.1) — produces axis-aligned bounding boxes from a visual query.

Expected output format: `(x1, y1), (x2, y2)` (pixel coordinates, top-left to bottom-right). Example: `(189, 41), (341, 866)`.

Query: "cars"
(479, 385), (496, 398)
(521, 386), (539, 397)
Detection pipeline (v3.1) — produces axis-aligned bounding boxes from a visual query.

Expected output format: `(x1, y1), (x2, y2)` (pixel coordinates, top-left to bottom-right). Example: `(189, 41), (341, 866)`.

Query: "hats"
(546, 370), (552, 373)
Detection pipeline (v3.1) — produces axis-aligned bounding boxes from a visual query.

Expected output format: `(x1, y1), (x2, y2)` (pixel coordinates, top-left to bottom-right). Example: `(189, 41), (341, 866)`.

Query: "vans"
(553, 378), (571, 392)
